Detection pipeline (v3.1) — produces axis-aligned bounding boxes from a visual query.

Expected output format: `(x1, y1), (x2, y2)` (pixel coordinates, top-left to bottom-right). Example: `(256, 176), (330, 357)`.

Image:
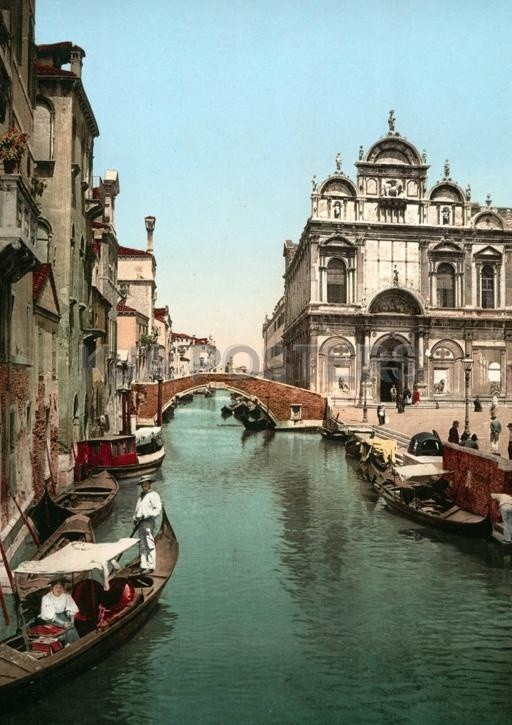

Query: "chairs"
(100, 578), (135, 621)
(71, 579), (104, 624)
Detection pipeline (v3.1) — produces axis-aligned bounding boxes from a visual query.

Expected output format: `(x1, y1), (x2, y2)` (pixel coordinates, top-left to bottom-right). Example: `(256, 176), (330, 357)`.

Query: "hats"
(136, 475), (157, 485)
(46, 573), (70, 585)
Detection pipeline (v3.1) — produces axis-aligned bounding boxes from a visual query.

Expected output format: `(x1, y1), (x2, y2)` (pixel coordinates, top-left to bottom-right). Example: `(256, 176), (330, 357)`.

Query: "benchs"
(17, 586), (68, 651)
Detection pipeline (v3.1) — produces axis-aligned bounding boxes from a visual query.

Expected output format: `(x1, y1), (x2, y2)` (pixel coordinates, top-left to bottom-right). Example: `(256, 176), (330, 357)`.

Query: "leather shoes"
(132, 568), (152, 575)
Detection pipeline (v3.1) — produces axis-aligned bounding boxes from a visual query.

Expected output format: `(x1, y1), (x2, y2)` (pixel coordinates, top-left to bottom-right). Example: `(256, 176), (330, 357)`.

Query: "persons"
(413, 386), (422, 408)
(309, 172), (319, 192)
(471, 433), (480, 449)
(460, 432), (471, 446)
(388, 108), (398, 131)
(334, 150), (345, 172)
(507, 423), (512, 462)
(472, 395), (484, 414)
(421, 147), (429, 163)
(444, 158), (452, 179)
(447, 419), (460, 445)
(38, 573), (82, 645)
(489, 417), (503, 453)
(389, 382), (398, 404)
(403, 386), (412, 404)
(465, 183), (473, 201)
(131, 473), (166, 576)
(358, 145), (364, 161)
(489, 391), (500, 420)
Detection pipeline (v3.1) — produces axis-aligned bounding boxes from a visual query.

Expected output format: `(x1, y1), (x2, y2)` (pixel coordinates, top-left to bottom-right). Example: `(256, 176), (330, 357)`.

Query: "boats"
(368, 458), (494, 539)
(24, 467), (120, 541)
(358, 439), (405, 484)
(13, 513), (98, 608)
(73, 434), (167, 484)
(343, 426), (376, 458)
(162, 387), (278, 431)
(319, 426), (351, 441)
(0, 489), (179, 700)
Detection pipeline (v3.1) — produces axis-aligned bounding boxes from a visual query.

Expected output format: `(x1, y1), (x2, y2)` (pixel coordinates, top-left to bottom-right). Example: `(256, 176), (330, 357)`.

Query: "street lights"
(361, 365), (372, 423)
(459, 353), (475, 439)
